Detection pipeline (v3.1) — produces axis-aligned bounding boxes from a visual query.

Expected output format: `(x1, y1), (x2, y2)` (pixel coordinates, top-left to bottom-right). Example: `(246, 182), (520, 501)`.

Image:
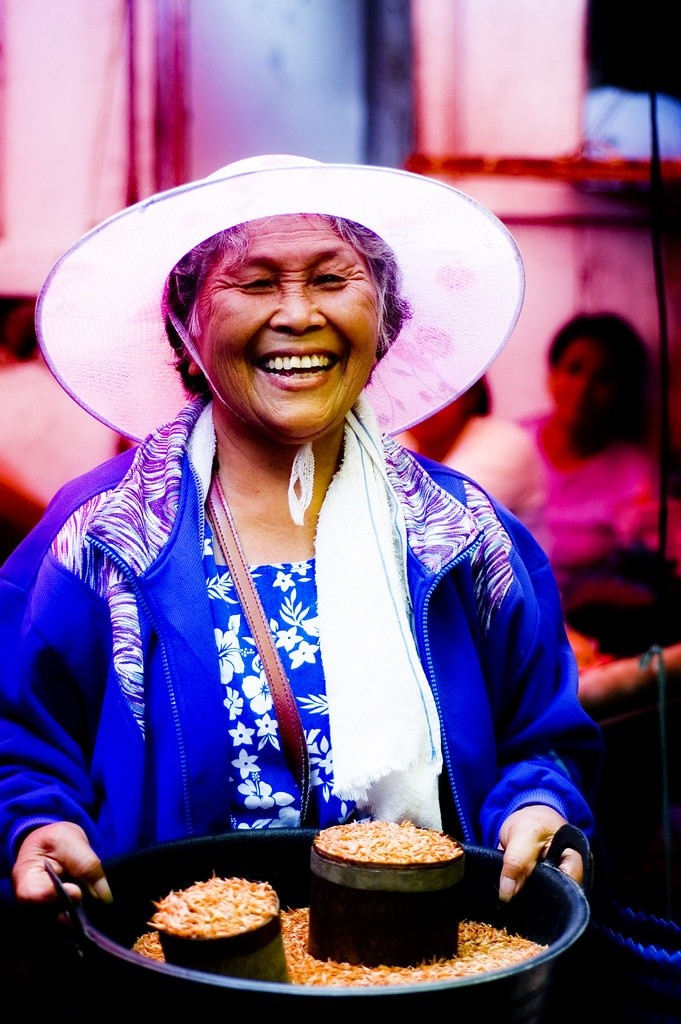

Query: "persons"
(514, 311), (681, 596)
(0, 154), (605, 1024)
(392, 371), (550, 577)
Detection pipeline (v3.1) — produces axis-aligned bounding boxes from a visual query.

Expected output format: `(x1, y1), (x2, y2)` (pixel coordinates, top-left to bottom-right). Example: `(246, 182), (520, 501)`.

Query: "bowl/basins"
(158, 881), (286, 985)
(45, 825), (591, 1024)
(308, 841), (466, 967)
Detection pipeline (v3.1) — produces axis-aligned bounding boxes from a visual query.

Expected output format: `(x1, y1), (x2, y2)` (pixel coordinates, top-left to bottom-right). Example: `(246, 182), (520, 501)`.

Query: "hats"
(37, 155), (524, 444)
